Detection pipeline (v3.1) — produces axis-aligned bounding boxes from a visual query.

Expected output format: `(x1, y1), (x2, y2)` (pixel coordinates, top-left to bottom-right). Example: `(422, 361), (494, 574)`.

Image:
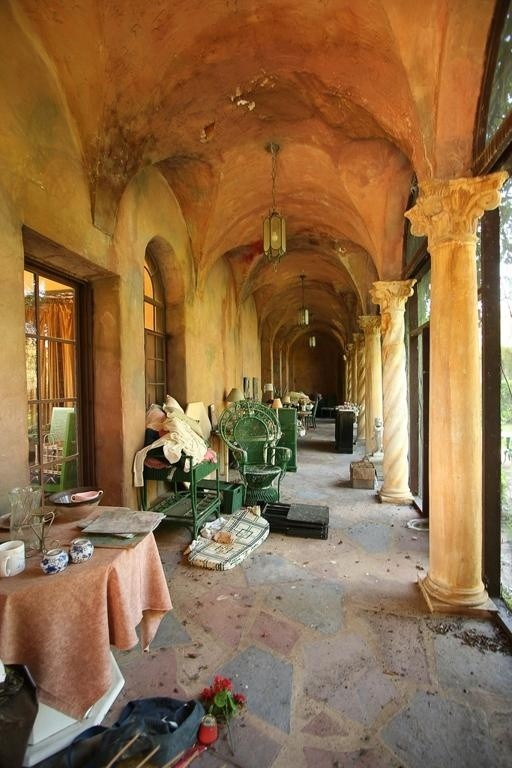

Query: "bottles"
(40, 537), (95, 575)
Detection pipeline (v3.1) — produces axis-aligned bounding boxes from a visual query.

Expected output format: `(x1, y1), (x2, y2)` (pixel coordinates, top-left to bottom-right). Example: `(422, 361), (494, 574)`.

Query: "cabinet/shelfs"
(140, 446), (222, 539)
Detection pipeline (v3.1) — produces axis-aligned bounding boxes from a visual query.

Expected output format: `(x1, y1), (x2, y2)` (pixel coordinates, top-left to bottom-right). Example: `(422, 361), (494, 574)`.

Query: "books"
(72, 507), (167, 550)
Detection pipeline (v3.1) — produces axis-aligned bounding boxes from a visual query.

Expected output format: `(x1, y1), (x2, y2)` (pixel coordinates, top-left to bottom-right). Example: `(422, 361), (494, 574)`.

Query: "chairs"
(217, 399), (293, 509)
(30, 405), (78, 493)
(303, 396), (318, 431)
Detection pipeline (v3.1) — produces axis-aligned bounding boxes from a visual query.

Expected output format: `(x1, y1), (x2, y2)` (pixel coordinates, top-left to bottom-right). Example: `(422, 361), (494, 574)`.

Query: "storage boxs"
(350, 460), (378, 490)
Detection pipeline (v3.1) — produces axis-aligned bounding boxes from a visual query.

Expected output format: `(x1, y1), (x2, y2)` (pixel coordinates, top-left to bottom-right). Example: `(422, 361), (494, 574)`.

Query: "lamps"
(296, 274), (317, 353)
(261, 143), (289, 275)
(226, 389), (291, 419)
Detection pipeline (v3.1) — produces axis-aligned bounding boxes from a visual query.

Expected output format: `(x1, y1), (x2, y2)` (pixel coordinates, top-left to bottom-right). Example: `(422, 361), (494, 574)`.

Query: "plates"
(0, 508), (55, 529)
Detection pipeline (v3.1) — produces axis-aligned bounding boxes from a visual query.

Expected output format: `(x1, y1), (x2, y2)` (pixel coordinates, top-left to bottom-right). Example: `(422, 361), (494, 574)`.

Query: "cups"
(70, 490), (103, 503)
(0, 540), (25, 578)
(6, 485), (45, 559)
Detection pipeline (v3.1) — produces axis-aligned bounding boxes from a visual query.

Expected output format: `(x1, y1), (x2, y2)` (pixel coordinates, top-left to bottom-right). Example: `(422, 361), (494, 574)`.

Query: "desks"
(297, 410), (312, 437)
(214, 429), (284, 486)
(0, 505), (153, 768)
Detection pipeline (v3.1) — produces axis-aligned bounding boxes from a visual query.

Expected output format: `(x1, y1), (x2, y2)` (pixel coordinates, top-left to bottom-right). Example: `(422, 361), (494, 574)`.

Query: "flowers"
(201, 677), (248, 757)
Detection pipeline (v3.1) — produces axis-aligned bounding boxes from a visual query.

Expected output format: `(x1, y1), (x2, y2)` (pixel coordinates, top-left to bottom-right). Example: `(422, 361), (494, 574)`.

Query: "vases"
(9, 487), (61, 559)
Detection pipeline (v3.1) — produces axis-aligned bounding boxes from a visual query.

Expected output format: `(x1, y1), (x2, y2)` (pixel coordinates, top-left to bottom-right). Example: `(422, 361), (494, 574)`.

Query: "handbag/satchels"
(66, 697), (207, 768)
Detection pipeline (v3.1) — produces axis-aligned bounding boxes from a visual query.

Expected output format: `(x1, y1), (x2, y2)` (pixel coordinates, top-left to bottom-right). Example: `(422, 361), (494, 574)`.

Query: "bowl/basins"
(48, 486), (104, 522)
